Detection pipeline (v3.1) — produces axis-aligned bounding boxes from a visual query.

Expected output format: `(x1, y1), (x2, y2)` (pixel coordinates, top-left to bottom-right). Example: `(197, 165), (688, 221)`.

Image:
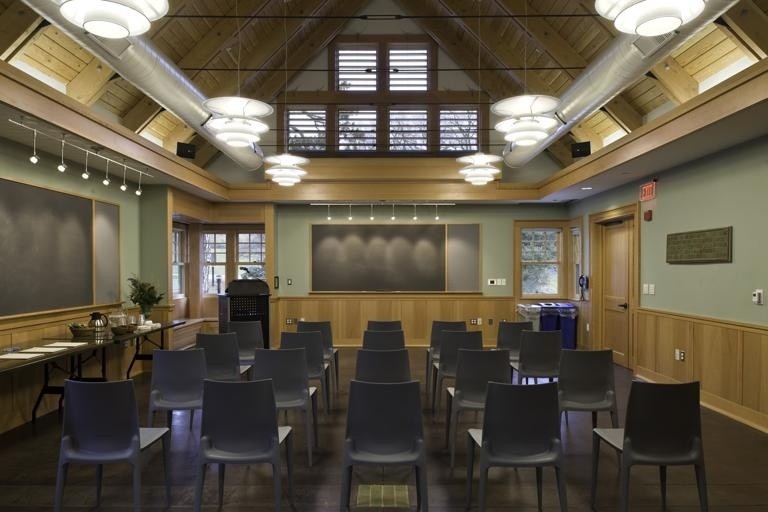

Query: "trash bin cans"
(516, 302), (578, 350)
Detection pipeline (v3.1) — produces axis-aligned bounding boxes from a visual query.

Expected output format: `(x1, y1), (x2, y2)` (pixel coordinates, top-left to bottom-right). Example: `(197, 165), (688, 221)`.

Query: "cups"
(129, 316), (136, 325)
(138, 315), (145, 328)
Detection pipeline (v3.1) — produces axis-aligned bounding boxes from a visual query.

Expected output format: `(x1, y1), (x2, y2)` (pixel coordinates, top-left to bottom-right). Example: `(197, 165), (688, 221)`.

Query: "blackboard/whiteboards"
(0, 176), (128, 320)
(307, 223), (482, 295)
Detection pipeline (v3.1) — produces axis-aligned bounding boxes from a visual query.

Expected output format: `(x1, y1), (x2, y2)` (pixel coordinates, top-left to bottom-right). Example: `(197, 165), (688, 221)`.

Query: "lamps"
(262, 0), (311, 187)
(595, 0), (709, 36)
(456, 0), (504, 185)
(49, 0), (169, 39)
(490, 0), (561, 146)
(8, 118), (153, 196)
(202, 0), (274, 148)
(311, 203), (455, 220)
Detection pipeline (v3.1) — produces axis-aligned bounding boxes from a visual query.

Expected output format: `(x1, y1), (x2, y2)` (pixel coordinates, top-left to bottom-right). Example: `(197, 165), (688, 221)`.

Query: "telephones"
(578, 275), (589, 290)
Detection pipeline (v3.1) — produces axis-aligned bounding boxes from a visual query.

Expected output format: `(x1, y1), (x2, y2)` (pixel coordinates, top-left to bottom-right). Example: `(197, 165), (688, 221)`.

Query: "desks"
(0, 320), (184, 424)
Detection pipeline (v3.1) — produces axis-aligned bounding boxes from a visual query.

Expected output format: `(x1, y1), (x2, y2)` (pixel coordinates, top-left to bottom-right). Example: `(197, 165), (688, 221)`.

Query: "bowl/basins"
(110, 324), (138, 336)
(69, 326), (95, 337)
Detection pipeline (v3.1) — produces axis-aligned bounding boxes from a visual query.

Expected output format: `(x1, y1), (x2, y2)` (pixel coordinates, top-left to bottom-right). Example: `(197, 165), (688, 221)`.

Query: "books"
(19, 346), (67, 353)
(0, 353), (45, 360)
(42, 341), (88, 348)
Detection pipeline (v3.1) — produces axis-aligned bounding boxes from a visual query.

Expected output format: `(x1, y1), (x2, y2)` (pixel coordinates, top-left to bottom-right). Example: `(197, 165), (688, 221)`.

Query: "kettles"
(88, 312), (109, 332)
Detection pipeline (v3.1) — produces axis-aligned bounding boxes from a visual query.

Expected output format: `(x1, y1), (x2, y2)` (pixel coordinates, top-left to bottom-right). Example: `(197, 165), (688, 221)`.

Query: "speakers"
(176, 141), (197, 158)
(571, 141), (591, 157)
